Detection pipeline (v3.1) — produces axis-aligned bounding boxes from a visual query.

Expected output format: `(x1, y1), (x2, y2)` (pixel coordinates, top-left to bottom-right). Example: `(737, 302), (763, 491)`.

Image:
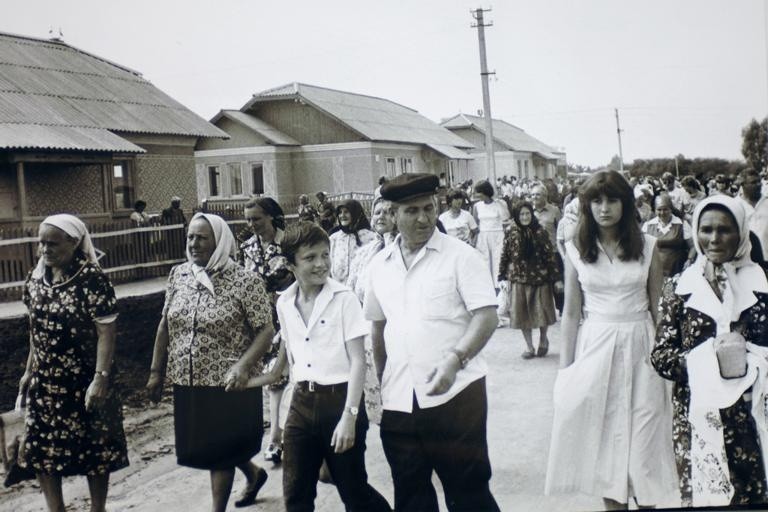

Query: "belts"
(298, 380), (347, 393)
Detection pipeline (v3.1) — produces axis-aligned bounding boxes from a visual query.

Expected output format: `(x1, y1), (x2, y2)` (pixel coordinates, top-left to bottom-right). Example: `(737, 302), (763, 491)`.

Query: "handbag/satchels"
(0, 383), (42, 473)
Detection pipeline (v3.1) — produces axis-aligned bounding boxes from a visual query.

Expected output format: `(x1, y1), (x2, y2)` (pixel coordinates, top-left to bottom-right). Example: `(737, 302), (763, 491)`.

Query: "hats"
(379, 172), (440, 202)
(171, 196), (180, 201)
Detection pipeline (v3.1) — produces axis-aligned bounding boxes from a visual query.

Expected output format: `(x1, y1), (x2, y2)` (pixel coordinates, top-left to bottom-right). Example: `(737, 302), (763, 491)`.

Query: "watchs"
(95, 370), (111, 378)
(343, 406), (359, 416)
(453, 349), (470, 369)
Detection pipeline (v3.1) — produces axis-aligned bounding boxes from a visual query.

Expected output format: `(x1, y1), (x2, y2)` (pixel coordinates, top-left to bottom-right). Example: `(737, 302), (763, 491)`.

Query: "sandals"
(538, 339), (548, 357)
(265, 428), (283, 460)
(236, 468), (267, 507)
(522, 348), (535, 359)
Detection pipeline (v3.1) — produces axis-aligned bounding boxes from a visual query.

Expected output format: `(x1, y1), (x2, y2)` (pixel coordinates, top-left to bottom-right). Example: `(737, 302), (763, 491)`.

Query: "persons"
(761, 174), (768, 197)
(320, 195), (335, 222)
(347, 196), (399, 290)
(498, 200), (564, 358)
(146, 211), (276, 512)
(643, 195), (696, 317)
(630, 172), (658, 221)
(682, 177), (705, 224)
(161, 196), (187, 259)
(459, 177), (470, 210)
(556, 197), (582, 263)
(449, 177), (454, 187)
(660, 172), (683, 210)
(131, 200), (152, 265)
(439, 189), (480, 244)
(497, 176), (532, 198)
(473, 180), (510, 295)
(545, 169), (682, 510)
(317, 192), (336, 229)
(298, 194), (319, 221)
(439, 172), (446, 187)
(466, 180), (473, 216)
(220, 221), (395, 512)
(237, 196), (295, 461)
(650, 193), (767, 512)
(328, 199), (384, 421)
(711, 174), (734, 198)
(544, 175), (579, 208)
(705, 177), (716, 195)
(738, 170), (768, 280)
(729, 173), (741, 195)
(18, 213), (130, 512)
(361, 173), (499, 512)
(564, 186), (578, 209)
(531, 185), (565, 317)
(374, 175), (390, 197)
(532, 176), (543, 186)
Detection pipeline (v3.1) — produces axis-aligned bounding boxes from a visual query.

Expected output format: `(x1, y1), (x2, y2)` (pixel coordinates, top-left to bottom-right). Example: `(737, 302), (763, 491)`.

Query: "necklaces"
(41, 253), (90, 301)
